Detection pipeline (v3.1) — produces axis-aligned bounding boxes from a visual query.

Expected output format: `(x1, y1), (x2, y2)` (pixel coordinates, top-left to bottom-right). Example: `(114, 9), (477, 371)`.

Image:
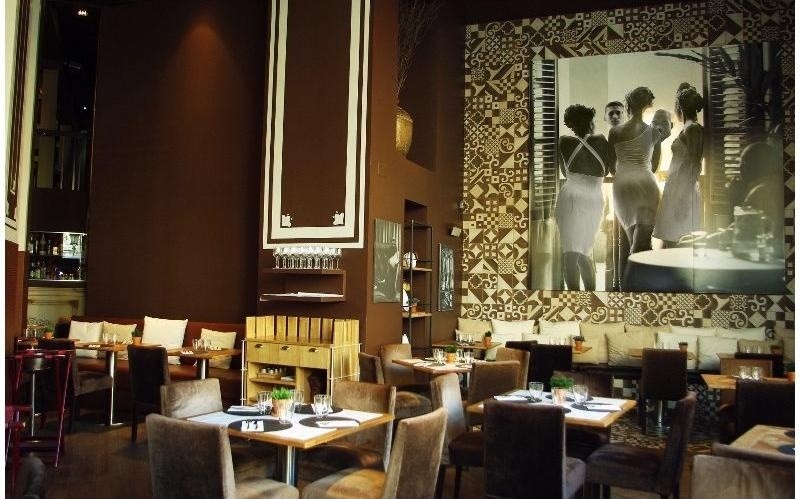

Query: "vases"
(393, 98), (414, 161)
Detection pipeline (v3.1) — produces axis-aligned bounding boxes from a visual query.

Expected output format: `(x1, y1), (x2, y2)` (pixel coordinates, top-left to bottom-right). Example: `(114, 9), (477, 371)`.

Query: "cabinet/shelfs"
(400, 217), (433, 361)
(260, 268), (347, 304)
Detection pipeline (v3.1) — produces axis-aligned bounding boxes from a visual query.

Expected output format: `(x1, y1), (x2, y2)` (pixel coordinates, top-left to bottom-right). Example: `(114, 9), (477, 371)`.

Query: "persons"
(603, 102), (626, 128)
(653, 82), (706, 247)
(653, 109), (672, 144)
(606, 86), (662, 292)
(559, 104), (611, 292)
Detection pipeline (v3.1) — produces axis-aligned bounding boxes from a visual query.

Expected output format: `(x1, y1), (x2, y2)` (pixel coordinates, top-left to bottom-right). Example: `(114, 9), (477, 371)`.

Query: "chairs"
(6, 307), (795, 498)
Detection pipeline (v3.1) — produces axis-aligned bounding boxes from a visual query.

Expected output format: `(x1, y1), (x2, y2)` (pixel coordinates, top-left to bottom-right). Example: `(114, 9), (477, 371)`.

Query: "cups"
(188, 337), (211, 353)
(25, 323), (39, 340)
(732, 200), (777, 262)
(529, 382), (544, 398)
(736, 365), (763, 382)
(252, 388), (338, 426)
(568, 385), (589, 407)
(101, 332), (117, 345)
(743, 343), (767, 355)
(431, 330), (477, 367)
(692, 232), (708, 258)
(551, 387), (564, 406)
(402, 258), (417, 267)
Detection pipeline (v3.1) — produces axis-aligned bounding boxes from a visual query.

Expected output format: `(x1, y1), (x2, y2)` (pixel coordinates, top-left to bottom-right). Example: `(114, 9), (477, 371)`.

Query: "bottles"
(258, 365), (289, 380)
(28, 235), (80, 279)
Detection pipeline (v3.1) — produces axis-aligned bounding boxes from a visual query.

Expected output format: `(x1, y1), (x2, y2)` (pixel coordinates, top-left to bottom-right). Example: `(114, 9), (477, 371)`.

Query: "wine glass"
(272, 245), (345, 271)
